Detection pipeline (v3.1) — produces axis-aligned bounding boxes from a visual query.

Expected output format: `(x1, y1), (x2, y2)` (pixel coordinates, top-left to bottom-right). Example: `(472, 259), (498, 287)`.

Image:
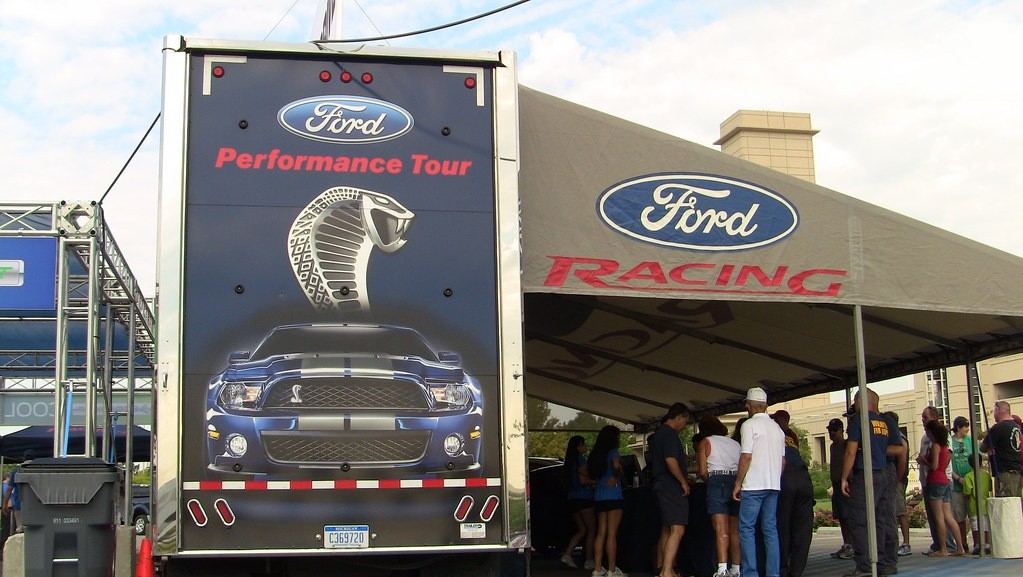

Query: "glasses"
(828, 428), (843, 432)
(995, 402), (1000, 410)
(959, 442), (964, 453)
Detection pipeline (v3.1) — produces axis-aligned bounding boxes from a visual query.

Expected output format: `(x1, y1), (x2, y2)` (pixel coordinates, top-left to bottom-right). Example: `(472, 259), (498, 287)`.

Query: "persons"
(687, 415), (741, 577)
(916, 401), (1023, 557)
(647, 402), (690, 577)
(586, 425), (629, 577)
(560, 436), (596, 569)
(826, 387), (912, 577)
(3, 467), (22, 533)
(732, 387), (786, 577)
(769, 410), (814, 577)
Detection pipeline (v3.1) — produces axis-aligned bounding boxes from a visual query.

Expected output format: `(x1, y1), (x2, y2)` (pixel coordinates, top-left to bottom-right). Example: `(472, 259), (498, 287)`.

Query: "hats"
(950, 416), (967, 431)
(743, 386), (767, 403)
(769, 410), (790, 419)
(842, 404), (857, 417)
(825, 418), (844, 429)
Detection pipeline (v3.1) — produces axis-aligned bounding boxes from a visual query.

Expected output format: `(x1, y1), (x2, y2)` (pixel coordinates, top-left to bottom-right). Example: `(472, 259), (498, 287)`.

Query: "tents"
(517, 84), (1023, 577)
(0, 425), (151, 465)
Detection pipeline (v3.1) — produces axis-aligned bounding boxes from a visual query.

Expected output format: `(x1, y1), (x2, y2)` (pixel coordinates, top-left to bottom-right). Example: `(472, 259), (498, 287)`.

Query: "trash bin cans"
(14, 457), (118, 577)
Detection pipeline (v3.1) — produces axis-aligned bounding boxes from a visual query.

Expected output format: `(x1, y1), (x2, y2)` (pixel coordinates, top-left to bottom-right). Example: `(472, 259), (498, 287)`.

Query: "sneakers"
(591, 566), (608, 577)
(898, 543), (912, 555)
(584, 560), (596, 569)
(607, 566), (628, 577)
(561, 554), (578, 569)
(840, 543), (855, 559)
(830, 545), (845, 558)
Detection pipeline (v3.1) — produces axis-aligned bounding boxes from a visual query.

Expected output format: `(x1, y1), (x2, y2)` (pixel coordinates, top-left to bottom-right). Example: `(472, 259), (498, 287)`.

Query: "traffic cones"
(133, 539), (156, 577)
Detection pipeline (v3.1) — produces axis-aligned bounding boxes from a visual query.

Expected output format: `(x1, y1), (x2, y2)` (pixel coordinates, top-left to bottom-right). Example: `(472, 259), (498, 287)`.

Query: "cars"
(131, 482), (150, 536)
(530, 455), (592, 560)
(199, 319), (489, 501)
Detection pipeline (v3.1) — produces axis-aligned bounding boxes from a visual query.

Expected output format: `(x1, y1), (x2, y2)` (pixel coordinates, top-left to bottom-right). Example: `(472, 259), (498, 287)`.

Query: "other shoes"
(843, 567), (898, 577)
(922, 548), (934, 555)
(712, 570), (732, 577)
